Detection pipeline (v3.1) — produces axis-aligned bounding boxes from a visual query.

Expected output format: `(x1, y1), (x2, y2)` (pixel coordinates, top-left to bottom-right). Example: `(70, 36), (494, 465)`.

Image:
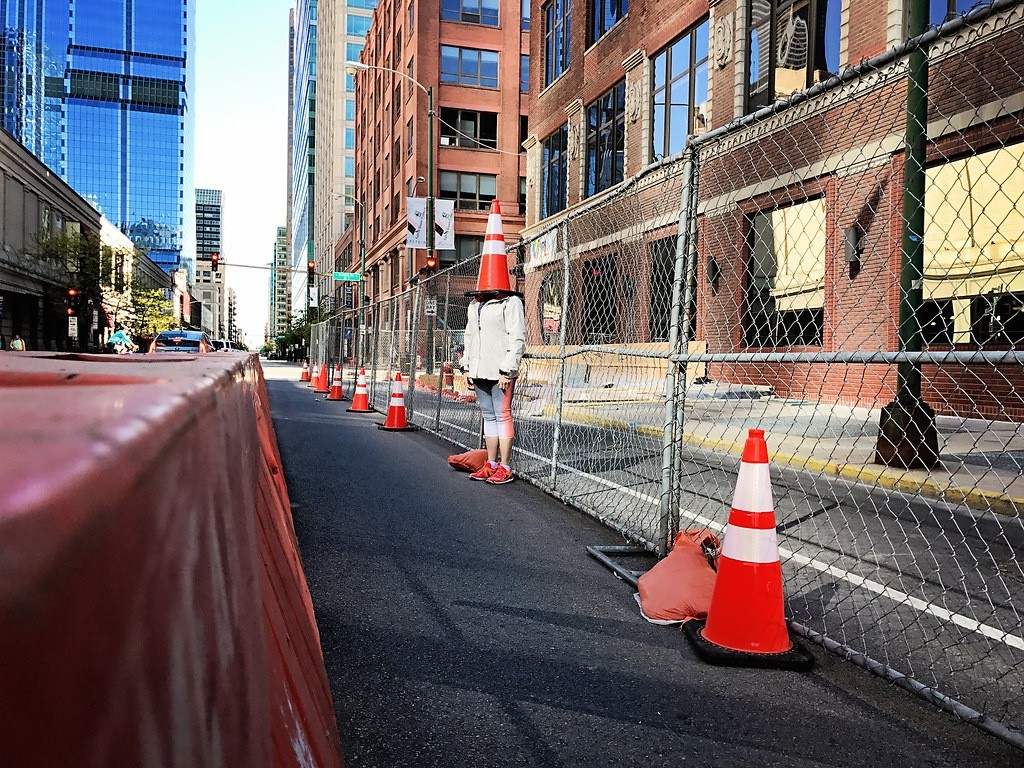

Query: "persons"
(10, 335), (25, 351)
(106, 326), (138, 355)
(459, 294), (526, 486)
(0, 335), (2, 350)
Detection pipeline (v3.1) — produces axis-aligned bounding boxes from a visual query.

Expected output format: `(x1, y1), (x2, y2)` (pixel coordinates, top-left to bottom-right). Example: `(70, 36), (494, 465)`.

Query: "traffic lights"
(307, 261), (314, 283)
(68, 287), (76, 308)
(426, 258), (437, 288)
(211, 253), (218, 272)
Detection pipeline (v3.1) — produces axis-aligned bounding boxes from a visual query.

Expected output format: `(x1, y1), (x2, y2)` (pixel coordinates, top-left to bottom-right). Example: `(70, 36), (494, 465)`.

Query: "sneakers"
(470, 462), (499, 481)
(486, 466), (514, 484)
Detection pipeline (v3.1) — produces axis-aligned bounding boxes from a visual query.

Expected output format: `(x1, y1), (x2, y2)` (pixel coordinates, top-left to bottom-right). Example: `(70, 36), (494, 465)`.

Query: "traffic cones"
(345, 368), (376, 413)
(461, 199), (524, 300)
(685, 427), (818, 671)
(314, 363), (331, 394)
(299, 360), (311, 382)
(306, 362), (320, 387)
(378, 372), (418, 432)
(324, 366), (347, 401)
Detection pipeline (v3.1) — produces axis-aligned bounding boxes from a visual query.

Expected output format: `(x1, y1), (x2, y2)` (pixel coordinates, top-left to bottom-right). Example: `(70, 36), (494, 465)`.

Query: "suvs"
(210, 340), (244, 352)
(147, 329), (222, 353)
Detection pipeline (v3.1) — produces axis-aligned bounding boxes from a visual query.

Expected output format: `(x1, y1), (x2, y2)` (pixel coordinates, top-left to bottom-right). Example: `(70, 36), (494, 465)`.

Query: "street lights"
(331, 190), (366, 368)
(339, 60), (437, 376)
(406, 176), (426, 373)
